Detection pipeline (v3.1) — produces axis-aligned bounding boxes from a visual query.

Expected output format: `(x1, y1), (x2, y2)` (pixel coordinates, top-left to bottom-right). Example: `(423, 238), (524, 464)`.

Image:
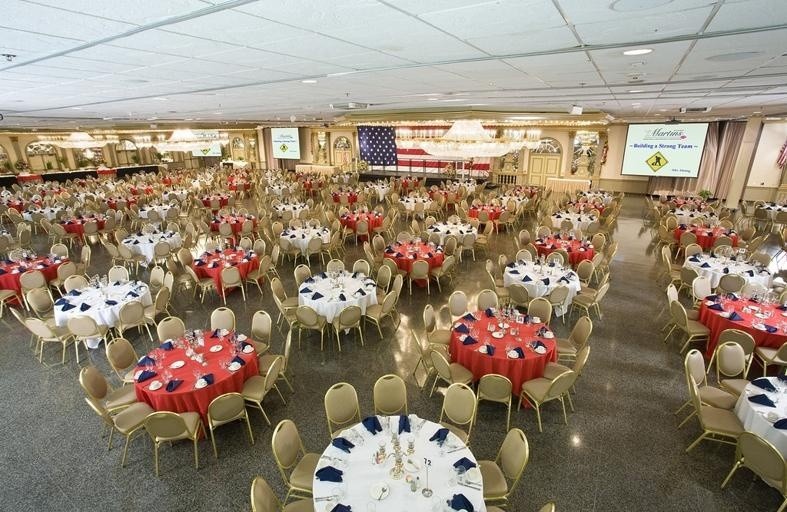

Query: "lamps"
(413, 119), (512, 159)
(160, 128), (203, 153)
(58, 131), (101, 151)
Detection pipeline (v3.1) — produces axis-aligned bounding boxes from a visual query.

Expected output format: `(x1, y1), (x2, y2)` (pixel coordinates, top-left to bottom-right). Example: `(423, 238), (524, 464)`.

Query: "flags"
(356, 123), (494, 178)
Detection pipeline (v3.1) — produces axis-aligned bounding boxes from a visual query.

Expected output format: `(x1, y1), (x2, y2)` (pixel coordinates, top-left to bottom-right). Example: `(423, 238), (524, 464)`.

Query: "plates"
(746, 374), (785, 421)
(312, 413), (483, 512)
(454, 308), (554, 357)
(134, 325), (256, 390)
(704, 292), (786, 334)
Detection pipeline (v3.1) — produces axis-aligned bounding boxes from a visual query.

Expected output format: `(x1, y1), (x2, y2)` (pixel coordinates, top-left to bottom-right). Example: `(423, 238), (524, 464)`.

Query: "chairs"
(0, 158), (787, 512)
